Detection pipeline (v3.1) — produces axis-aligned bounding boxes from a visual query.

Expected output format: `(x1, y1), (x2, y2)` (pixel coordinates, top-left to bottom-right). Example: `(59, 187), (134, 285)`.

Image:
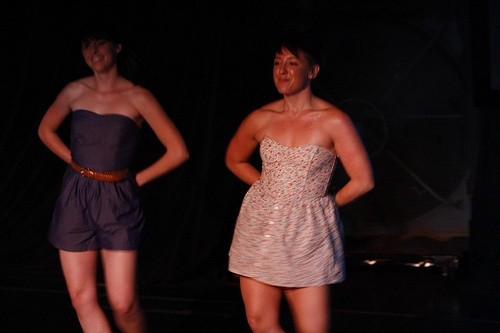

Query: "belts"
(71, 159), (128, 182)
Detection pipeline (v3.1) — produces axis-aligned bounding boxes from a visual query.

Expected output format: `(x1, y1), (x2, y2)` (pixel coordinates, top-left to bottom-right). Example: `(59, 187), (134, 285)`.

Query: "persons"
(225, 26), (376, 333)
(38, 24), (191, 333)
